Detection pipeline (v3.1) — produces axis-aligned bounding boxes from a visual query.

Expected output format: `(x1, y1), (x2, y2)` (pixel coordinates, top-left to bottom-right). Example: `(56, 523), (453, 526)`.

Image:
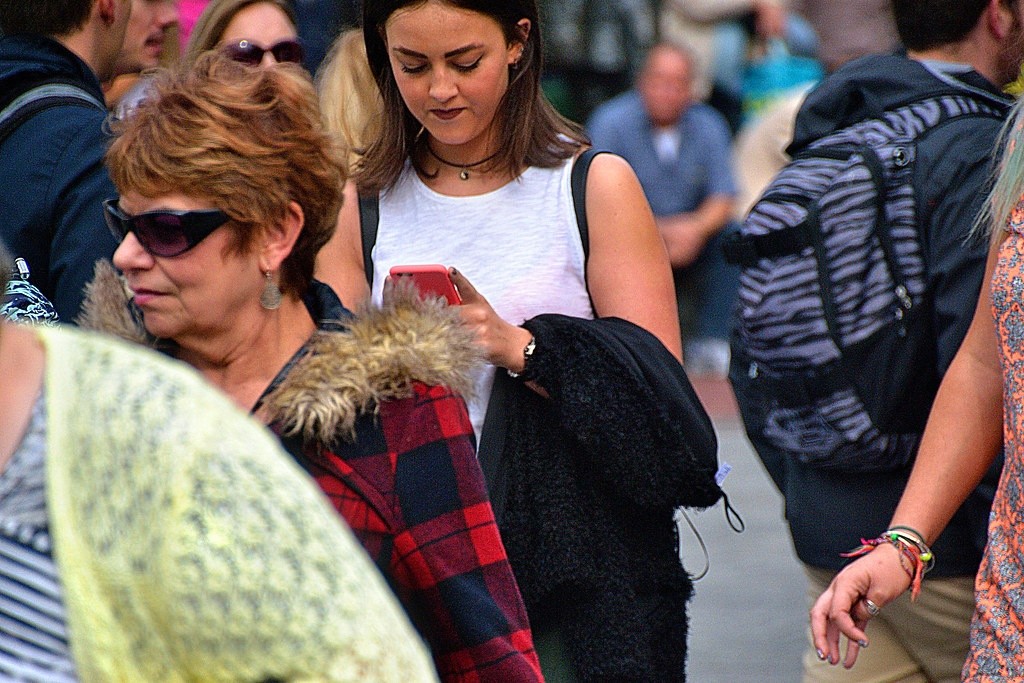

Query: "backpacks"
(718, 93), (1018, 478)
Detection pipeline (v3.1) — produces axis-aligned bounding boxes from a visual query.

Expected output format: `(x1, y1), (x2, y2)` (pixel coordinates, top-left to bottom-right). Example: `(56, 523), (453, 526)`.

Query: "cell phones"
(390, 264), (460, 307)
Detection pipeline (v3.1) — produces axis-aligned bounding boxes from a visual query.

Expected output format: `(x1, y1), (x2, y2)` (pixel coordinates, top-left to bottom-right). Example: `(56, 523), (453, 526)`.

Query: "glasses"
(212, 38), (304, 69)
(102, 198), (238, 258)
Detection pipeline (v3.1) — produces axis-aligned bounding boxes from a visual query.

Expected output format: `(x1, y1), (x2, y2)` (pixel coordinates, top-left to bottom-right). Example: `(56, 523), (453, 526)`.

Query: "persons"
(0, 0), (1024, 683)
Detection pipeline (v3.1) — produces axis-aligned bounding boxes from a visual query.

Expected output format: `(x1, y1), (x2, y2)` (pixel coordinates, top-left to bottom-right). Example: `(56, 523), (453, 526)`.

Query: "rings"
(864, 599), (880, 616)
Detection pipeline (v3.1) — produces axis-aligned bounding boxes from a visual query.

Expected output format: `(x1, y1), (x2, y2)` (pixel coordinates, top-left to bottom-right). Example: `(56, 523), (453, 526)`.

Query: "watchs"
(507, 338), (536, 381)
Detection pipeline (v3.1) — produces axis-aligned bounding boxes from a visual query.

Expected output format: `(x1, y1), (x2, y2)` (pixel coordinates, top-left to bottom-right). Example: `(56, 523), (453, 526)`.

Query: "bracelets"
(846, 525), (935, 596)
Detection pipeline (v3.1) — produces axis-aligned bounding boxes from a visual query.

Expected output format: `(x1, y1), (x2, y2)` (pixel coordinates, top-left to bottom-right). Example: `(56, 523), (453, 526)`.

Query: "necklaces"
(427, 142), (506, 179)
